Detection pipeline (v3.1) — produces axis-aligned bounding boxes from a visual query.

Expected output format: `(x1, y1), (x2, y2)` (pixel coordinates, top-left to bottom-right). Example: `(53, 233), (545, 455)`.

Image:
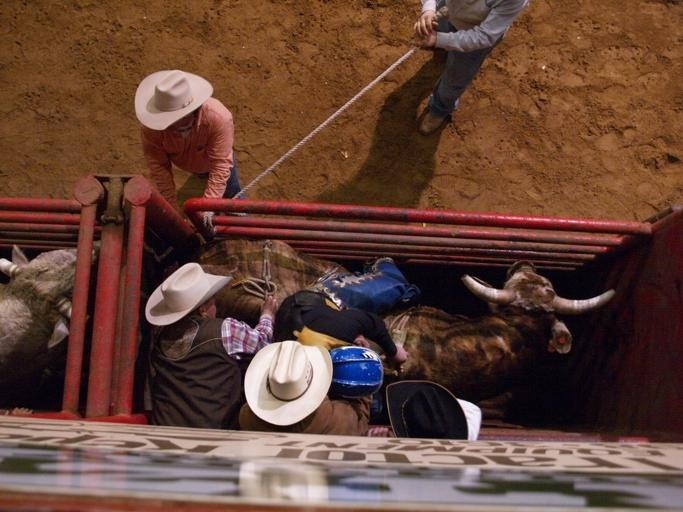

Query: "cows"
(198, 239), (615, 400)
(0, 244), (96, 361)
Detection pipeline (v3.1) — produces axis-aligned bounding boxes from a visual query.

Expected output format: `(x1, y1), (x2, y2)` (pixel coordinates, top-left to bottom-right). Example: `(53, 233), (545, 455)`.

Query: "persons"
(271, 255), (421, 400)
(411, 1), (530, 137)
(132, 67), (246, 221)
(385, 380), (483, 442)
(237, 337), (373, 434)
(140, 260), (284, 431)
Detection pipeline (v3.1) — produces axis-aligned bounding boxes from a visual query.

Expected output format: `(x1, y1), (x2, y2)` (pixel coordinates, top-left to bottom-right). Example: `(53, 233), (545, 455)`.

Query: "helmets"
(331, 346), (383, 399)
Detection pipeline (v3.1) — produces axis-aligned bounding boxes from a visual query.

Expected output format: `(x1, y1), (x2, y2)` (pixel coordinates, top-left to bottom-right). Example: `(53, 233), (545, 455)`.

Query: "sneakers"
(419, 111), (446, 137)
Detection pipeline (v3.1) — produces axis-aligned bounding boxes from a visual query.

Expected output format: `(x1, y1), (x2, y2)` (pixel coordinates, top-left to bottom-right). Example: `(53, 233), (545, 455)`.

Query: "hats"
(385, 379), (469, 440)
(243, 339), (334, 427)
(144, 262), (233, 327)
(134, 70), (214, 131)
